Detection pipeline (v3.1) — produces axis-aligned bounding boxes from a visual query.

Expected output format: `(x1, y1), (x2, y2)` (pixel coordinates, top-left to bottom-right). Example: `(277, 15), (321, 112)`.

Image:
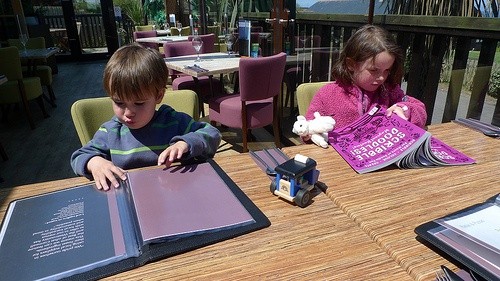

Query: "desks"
(0, 148), (413, 281)
(18, 48), (56, 77)
(164, 51), (242, 130)
(49, 27), (67, 51)
(157, 30), (171, 34)
(136, 35), (189, 43)
(283, 120), (500, 281)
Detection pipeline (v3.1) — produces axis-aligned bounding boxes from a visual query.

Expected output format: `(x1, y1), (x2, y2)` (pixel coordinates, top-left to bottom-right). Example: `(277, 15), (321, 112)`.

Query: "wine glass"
(191, 35), (205, 63)
(177, 23), (182, 37)
(164, 27), (170, 38)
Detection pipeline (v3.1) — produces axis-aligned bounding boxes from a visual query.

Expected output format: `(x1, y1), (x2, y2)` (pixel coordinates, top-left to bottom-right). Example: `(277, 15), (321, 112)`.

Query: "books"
(329, 103), (477, 175)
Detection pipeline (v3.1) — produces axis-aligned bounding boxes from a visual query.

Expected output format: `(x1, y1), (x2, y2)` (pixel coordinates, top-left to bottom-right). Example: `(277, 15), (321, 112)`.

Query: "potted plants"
(35, 5), (48, 25)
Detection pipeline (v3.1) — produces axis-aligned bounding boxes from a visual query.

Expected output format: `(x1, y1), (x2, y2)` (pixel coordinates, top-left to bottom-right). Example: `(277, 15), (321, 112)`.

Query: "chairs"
(70, 90), (200, 147)
(8, 37), (55, 109)
(134, 26), (287, 153)
(297, 81), (335, 117)
(0, 46), (48, 129)
(63, 22), (83, 51)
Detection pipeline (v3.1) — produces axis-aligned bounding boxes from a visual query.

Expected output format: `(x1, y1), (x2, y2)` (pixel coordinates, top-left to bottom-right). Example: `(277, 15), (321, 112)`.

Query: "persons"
(70, 44), (222, 190)
(299, 24), (427, 145)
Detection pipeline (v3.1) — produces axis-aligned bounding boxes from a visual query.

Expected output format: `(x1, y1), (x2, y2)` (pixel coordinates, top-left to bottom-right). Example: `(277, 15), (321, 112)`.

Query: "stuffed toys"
(292, 112), (336, 149)
